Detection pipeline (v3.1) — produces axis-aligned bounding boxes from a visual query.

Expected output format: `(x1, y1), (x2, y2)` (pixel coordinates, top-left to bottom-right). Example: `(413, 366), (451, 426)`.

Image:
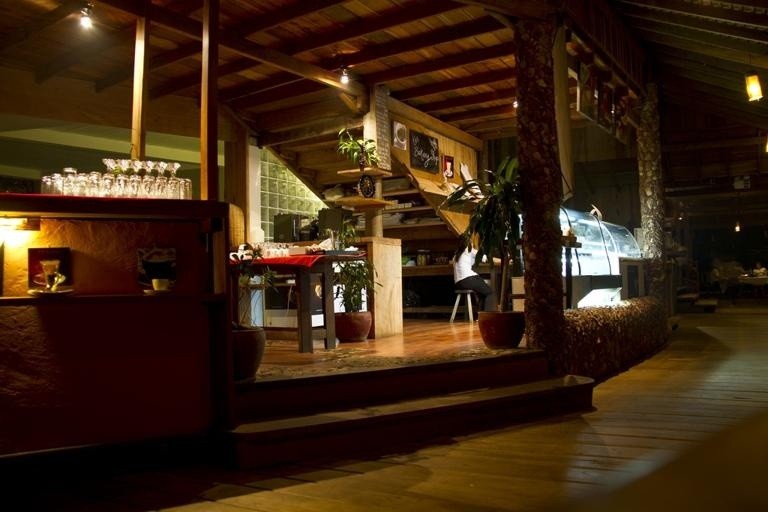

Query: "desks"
(230, 252), (366, 353)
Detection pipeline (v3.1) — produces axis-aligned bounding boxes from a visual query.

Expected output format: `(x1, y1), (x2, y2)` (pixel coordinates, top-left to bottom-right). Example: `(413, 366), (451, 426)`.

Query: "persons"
(710, 264), (720, 284)
(753, 262), (765, 275)
(453, 233), (493, 312)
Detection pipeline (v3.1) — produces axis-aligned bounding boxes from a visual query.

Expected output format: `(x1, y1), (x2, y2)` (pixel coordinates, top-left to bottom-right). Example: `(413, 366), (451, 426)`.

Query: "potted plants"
(447, 157), (525, 349)
(232, 244), (278, 384)
(339, 128), (382, 167)
(318, 218), (379, 343)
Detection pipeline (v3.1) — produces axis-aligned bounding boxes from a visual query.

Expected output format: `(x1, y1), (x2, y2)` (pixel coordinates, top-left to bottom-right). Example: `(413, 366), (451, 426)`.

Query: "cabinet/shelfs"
(261, 146), (329, 242)
(512, 205), (642, 311)
(325, 189), (445, 230)
(402, 263), (511, 313)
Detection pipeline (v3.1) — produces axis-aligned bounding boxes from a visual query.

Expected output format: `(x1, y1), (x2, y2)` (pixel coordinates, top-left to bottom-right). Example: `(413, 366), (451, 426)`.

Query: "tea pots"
(237, 243), (254, 260)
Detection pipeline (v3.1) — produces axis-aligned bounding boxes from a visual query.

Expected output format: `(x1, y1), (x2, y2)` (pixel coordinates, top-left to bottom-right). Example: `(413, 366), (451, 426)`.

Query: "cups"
(252, 243), (290, 258)
(40, 259), (60, 288)
(180, 178), (192, 200)
(40, 166), (102, 196)
(152, 278), (169, 291)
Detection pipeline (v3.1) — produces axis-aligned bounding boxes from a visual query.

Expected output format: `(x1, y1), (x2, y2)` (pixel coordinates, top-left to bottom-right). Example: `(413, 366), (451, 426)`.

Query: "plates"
(144, 289), (171, 294)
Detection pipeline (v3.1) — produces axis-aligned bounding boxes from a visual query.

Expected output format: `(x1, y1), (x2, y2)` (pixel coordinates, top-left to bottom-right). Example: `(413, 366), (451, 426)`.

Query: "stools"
(450, 289), (481, 325)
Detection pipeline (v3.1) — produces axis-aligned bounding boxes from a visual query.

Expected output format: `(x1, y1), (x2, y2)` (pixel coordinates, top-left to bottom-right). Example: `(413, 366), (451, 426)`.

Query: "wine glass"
(102, 158), (180, 198)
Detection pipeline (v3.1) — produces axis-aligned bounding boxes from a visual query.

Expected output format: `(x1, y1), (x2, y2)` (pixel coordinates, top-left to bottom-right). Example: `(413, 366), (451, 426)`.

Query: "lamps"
(338, 51), (349, 84)
(744, 42), (764, 102)
(81, 3), (94, 27)
